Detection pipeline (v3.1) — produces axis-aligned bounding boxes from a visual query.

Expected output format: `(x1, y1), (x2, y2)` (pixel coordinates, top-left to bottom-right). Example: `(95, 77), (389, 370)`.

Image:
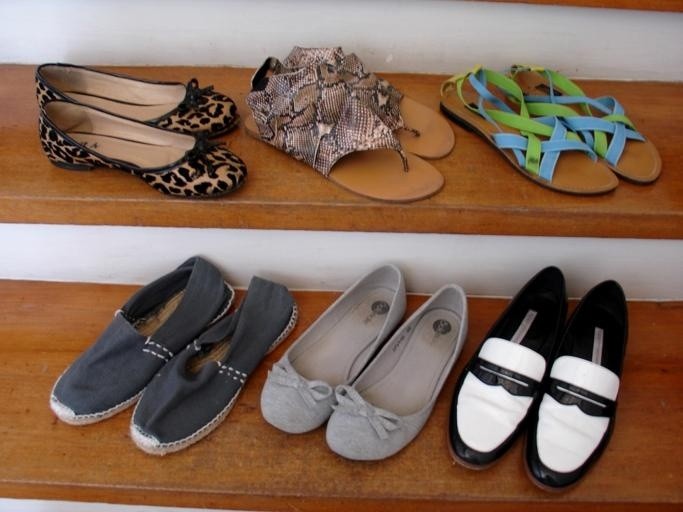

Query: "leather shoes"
(445, 266), (570, 471)
(526, 279), (629, 493)
(326, 283), (472, 463)
(33, 57), (241, 135)
(256, 260), (407, 436)
(38, 100), (246, 198)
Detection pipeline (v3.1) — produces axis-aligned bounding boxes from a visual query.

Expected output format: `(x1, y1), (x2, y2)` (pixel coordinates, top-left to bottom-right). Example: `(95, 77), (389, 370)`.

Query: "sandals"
(289, 41), (456, 160)
(241, 53), (444, 204)
(502, 62), (664, 186)
(439, 65), (619, 196)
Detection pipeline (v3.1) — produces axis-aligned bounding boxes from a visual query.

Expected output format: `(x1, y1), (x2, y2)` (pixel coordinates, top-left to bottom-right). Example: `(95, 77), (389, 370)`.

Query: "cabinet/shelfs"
(0, 61), (682, 512)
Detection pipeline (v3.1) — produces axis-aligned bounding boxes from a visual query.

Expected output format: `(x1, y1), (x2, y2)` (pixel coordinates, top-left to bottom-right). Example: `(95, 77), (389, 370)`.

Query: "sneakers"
(128, 275), (295, 457)
(48, 254), (235, 427)
